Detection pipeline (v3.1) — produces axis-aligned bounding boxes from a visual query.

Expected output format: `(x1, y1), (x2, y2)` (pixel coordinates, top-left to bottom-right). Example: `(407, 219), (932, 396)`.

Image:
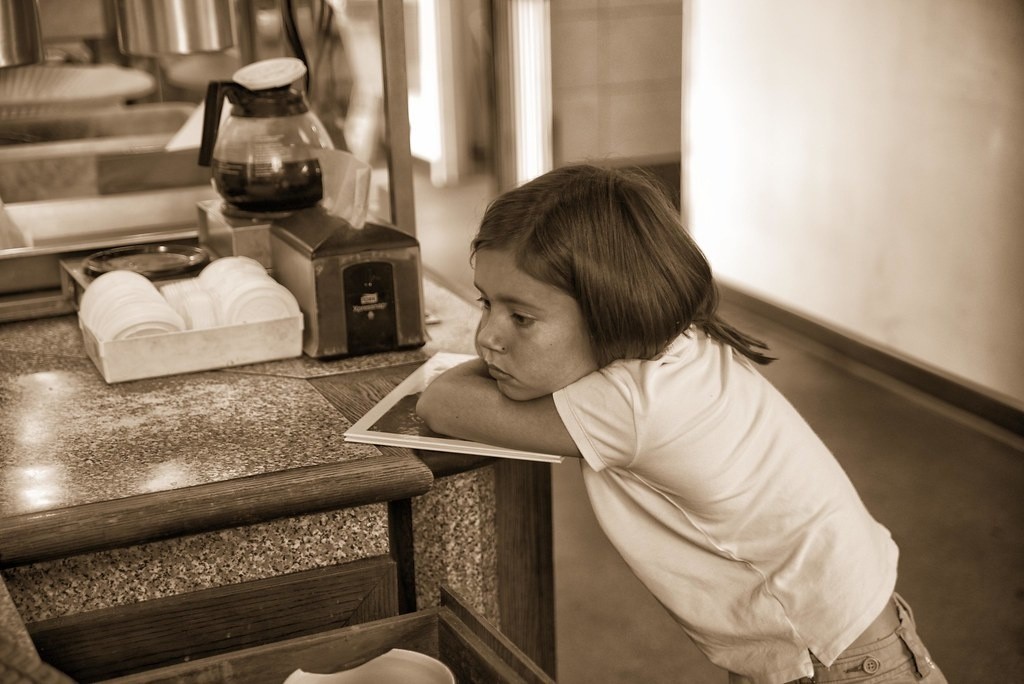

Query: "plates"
(84, 244), (208, 279)
(83, 260), (302, 339)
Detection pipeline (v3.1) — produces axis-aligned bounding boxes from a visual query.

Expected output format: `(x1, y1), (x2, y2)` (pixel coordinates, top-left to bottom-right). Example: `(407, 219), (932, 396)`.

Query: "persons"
(414, 163), (950, 683)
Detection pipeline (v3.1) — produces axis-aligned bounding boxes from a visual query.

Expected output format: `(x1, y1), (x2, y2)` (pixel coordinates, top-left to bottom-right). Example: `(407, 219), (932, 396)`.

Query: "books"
(345, 351), (565, 464)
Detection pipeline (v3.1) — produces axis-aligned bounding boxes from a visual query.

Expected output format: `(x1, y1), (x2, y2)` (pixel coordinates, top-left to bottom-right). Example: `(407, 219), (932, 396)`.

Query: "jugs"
(197, 57), (338, 218)
